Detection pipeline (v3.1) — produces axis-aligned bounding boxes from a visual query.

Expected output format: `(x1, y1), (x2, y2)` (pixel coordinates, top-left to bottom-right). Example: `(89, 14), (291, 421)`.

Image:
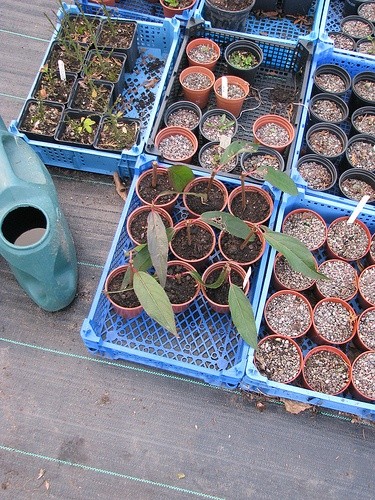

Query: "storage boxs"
(73, 0), (200, 35)
(80, 153), (282, 391)
(239, 187), (375, 422)
(318, 0), (375, 69)
(190, 0), (324, 71)
(144, 17), (316, 185)
(9, 1), (181, 177)
(289, 42), (375, 210)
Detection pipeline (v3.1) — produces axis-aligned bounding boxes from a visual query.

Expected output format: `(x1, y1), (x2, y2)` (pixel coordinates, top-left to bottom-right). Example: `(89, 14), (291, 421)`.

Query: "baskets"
(144, 18), (315, 187)
(78, 0), (201, 47)
(192, 0), (324, 69)
(80, 154), (283, 390)
(9, 0), (182, 178)
(238, 188), (375, 422)
(290, 41), (375, 212)
(318, 0), (375, 62)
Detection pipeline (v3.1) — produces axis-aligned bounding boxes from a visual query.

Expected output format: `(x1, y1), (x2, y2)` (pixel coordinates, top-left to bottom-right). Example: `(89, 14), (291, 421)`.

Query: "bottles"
(0, 116), (78, 311)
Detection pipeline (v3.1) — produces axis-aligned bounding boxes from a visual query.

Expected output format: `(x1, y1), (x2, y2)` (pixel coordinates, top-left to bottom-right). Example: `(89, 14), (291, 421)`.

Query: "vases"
(253, 208), (375, 405)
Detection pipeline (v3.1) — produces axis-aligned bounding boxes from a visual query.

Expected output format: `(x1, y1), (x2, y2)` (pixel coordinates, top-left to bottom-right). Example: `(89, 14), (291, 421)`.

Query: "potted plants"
(155, 37), (295, 181)
(296, 63), (375, 206)
(103, 164), (330, 352)
(206, 0), (316, 32)
(328, 0), (375, 56)
(17, 13), (140, 154)
